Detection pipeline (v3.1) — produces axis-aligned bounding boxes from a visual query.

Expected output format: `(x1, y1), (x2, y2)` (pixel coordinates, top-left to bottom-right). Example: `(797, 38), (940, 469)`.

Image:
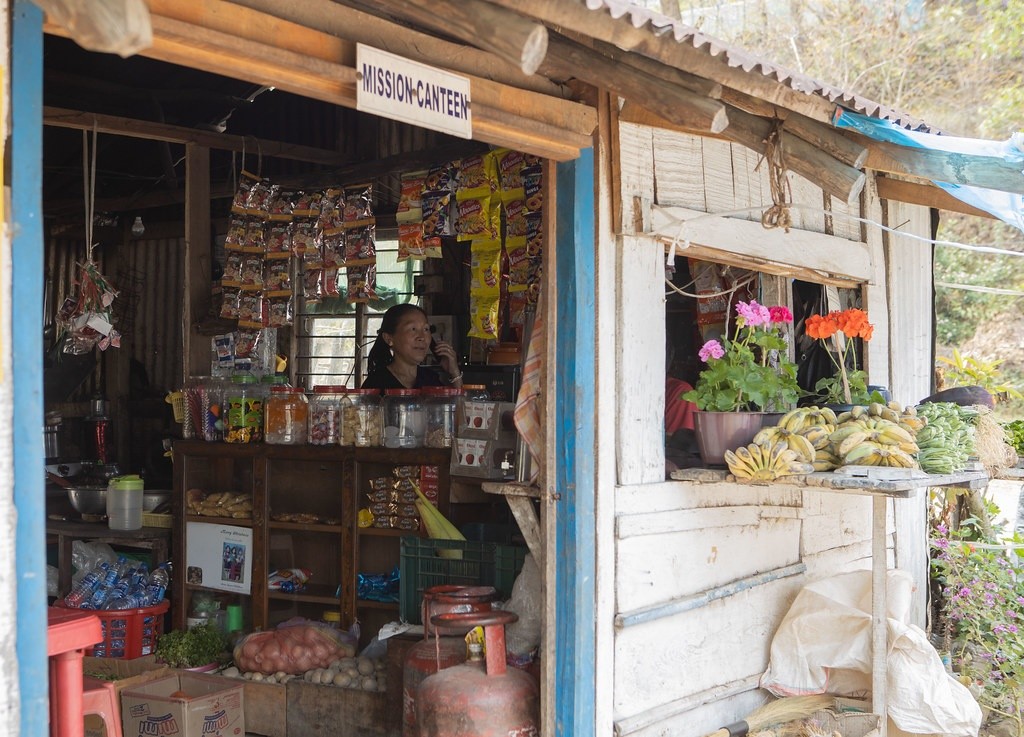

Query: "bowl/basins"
(65, 486), (108, 515)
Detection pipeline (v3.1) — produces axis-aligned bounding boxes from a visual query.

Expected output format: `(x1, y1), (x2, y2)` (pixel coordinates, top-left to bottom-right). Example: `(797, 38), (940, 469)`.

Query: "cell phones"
(429, 336), (438, 355)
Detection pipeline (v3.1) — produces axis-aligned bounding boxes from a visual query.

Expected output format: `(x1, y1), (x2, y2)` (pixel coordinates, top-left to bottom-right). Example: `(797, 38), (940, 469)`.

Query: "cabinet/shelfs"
(171, 440), (494, 639)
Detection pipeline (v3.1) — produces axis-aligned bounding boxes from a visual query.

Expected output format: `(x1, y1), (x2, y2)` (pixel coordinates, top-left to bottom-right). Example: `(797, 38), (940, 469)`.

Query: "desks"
(47, 498), (177, 609)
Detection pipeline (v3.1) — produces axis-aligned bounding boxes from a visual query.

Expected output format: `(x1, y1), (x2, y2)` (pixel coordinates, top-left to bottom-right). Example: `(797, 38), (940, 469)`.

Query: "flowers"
(754, 306), (802, 412)
(805, 308), (886, 405)
(680, 299), (778, 413)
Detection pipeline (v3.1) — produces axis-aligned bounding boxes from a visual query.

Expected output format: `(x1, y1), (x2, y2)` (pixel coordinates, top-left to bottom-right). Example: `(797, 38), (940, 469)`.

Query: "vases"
(759, 411), (787, 427)
(820, 403), (870, 417)
(691, 411), (764, 470)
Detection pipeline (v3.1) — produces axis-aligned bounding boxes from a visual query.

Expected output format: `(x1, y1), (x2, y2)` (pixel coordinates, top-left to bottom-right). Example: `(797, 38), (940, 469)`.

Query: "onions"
(224, 618), (387, 692)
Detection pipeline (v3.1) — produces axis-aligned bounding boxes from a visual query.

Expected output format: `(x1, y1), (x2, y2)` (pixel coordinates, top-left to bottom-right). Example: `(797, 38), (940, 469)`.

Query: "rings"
(447, 349), (451, 352)
(447, 344), (453, 350)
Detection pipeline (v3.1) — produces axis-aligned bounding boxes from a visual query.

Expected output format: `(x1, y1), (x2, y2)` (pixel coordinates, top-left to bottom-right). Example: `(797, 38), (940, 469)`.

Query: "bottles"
(66, 555), (170, 660)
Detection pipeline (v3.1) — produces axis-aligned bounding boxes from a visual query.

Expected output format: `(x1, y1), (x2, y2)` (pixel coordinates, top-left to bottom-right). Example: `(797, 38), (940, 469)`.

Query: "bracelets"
(447, 370), (464, 384)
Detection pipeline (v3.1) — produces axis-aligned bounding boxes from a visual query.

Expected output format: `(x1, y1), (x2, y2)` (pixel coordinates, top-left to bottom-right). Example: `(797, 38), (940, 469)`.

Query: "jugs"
(106, 472), (144, 531)
(82, 398), (115, 465)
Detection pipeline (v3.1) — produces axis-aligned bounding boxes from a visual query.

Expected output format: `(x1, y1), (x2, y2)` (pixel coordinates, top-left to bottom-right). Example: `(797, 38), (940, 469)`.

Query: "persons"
(187, 564), (203, 584)
(233, 548), (245, 583)
(359, 301), (466, 394)
(227, 547), (239, 582)
(223, 544), (232, 580)
(664, 330), (707, 481)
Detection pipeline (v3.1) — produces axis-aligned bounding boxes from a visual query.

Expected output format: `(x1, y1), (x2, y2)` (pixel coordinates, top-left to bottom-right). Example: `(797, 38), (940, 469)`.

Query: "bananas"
(723, 399), (925, 480)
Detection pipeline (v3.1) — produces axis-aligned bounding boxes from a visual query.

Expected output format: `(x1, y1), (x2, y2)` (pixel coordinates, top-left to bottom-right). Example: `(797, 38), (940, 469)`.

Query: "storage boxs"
(53, 598), (383, 737)
(398, 537), (531, 628)
